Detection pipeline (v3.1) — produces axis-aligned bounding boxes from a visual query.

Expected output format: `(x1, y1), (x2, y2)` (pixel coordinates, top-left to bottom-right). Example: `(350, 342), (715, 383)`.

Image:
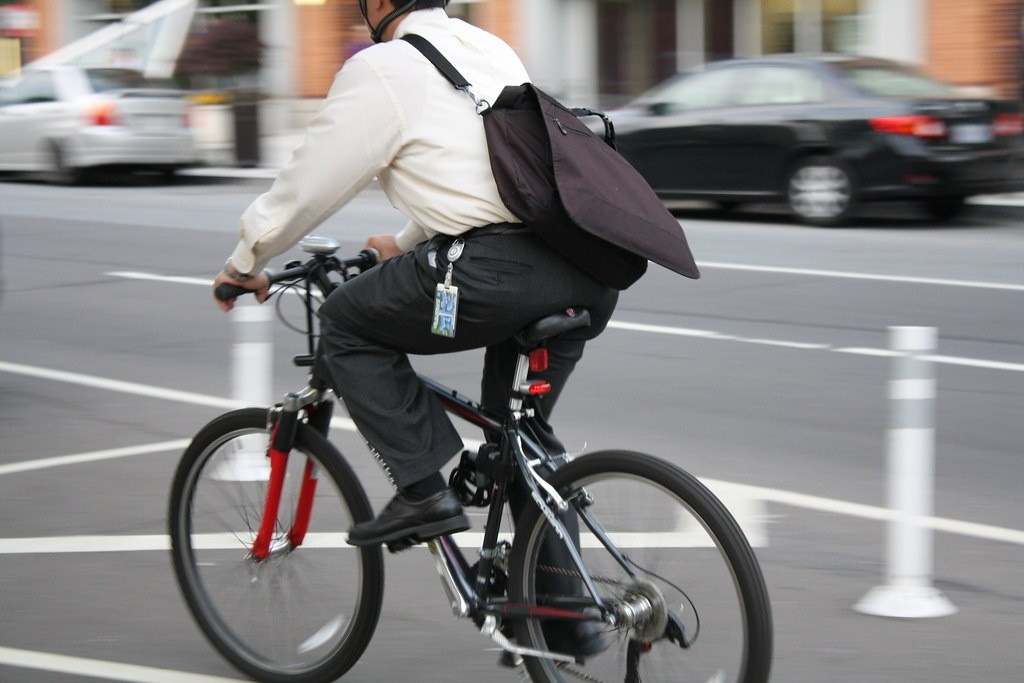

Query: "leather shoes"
(346, 493), (471, 549)
(500, 605), (620, 653)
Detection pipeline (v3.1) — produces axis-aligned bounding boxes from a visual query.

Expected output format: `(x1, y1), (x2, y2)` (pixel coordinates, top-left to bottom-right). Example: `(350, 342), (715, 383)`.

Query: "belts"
(465, 225), (528, 238)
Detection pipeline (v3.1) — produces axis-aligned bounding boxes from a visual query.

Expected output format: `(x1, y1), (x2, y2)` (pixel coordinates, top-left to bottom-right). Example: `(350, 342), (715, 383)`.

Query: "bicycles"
(167, 233), (775, 683)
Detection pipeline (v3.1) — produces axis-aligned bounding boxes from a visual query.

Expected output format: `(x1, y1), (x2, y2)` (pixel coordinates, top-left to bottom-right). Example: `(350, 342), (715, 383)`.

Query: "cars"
(577, 49), (1024, 230)
(0, 58), (212, 189)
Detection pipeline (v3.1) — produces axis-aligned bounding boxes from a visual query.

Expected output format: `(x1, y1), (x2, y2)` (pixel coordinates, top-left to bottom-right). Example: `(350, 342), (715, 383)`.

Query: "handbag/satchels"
(397, 32), (705, 294)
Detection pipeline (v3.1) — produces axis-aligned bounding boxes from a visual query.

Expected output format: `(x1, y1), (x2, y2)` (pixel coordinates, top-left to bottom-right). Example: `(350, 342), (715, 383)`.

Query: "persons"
(213, 0), (619, 658)
